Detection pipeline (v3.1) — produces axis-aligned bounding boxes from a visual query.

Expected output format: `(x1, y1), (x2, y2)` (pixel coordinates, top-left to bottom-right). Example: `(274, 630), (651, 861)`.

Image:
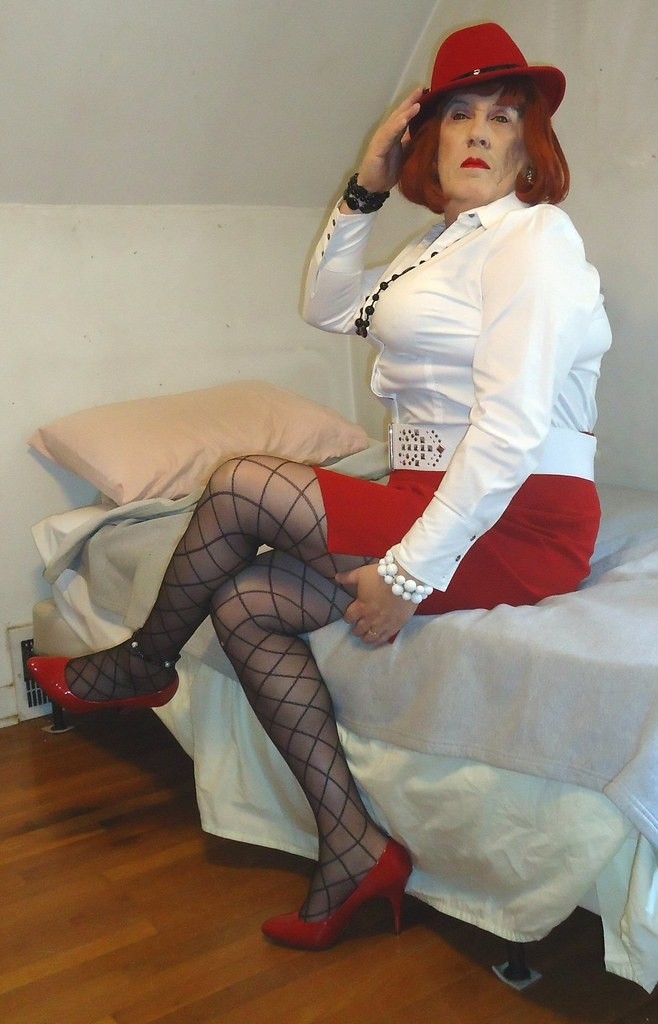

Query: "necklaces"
(355, 236), (480, 338)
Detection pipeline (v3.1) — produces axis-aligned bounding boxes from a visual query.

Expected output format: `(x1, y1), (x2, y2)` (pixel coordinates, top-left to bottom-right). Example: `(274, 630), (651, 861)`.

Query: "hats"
(408, 23), (566, 140)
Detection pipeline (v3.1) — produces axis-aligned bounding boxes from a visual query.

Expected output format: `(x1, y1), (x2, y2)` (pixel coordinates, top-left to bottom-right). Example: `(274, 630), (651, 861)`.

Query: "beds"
(28, 442), (657, 999)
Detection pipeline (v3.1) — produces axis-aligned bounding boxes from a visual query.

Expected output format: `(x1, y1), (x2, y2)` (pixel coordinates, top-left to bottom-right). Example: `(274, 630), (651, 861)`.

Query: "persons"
(28, 23), (611, 950)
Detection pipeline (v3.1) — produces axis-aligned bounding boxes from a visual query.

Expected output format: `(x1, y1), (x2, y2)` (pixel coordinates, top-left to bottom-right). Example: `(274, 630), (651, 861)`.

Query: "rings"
(368, 629), (380, 637)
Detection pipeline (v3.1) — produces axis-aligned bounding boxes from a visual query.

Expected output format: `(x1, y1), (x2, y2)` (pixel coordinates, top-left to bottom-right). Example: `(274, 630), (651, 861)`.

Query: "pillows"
(29, 382), (368, 507)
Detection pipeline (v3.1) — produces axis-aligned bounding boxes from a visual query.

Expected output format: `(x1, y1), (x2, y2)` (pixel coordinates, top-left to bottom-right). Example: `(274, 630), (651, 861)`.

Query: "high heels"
(260, 837), (413, 952)
(26, 656), (180, 717)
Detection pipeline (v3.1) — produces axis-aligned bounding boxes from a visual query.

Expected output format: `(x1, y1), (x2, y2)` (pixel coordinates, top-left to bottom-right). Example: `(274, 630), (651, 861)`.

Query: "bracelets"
(377, 550), (433, 602)
(342, 171), (393, 214)
